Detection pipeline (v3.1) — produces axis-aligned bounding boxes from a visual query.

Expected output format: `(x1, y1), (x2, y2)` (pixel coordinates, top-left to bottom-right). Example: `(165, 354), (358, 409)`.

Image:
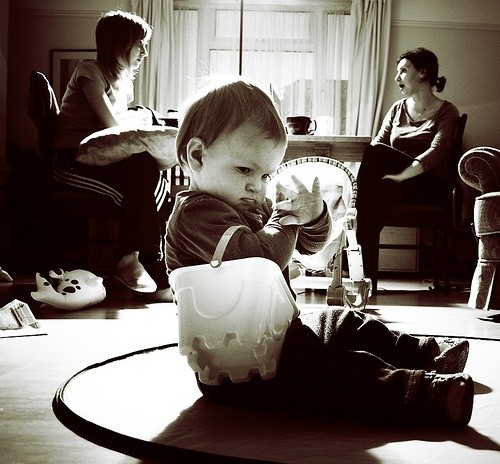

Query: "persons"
(162, 82), (475, 428)
(342, 47), (461, 270)
(47, 9), (174, 302)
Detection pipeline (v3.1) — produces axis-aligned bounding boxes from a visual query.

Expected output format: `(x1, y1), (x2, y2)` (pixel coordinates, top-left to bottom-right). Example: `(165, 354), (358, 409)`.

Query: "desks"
(282, 134), (421, 281)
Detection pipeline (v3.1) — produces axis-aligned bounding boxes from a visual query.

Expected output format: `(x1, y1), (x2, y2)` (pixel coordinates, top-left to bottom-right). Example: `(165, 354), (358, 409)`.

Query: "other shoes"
(419, 372), (475, 429)
(422, 337), (468, 371)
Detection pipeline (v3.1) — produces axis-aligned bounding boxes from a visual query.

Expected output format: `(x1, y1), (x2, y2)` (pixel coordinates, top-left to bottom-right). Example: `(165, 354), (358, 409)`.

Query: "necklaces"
(415, 95), (432, 119)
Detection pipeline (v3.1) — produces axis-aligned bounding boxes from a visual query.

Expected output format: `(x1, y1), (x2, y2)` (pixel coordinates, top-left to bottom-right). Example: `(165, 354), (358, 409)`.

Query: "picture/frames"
(50, 47), (100, 106)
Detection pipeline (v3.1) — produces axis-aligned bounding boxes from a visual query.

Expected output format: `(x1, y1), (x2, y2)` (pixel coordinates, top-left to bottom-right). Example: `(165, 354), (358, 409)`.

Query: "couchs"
(458, 146), (500, 312)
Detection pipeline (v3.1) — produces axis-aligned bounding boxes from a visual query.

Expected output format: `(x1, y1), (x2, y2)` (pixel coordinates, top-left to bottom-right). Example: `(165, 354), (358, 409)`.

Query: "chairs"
(373, 112), (468, 292)
(29, 70), (123, 277)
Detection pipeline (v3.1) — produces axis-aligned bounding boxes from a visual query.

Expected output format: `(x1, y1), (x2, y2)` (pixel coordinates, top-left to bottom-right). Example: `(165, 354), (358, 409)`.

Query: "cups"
(286, 116), (317, 135)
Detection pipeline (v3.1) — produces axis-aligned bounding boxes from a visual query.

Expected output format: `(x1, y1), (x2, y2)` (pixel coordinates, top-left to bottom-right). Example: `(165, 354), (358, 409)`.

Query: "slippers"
(114, 261), (157, 293)
(143, 286), (172, 302)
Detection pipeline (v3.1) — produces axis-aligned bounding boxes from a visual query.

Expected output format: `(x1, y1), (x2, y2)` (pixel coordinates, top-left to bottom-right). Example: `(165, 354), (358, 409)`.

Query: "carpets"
(52, 332), (500, 464)
